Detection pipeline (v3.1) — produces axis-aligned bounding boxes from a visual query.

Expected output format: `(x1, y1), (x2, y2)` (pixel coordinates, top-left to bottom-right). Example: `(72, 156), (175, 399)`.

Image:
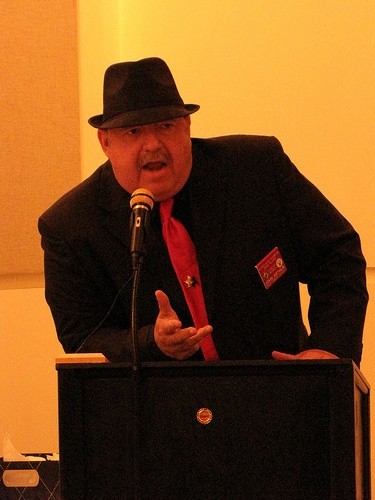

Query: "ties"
(160, 198), (218, 361)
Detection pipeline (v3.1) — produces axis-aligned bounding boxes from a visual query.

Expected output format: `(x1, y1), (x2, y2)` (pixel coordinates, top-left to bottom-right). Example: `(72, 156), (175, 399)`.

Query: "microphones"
(129, 188), (154, 271)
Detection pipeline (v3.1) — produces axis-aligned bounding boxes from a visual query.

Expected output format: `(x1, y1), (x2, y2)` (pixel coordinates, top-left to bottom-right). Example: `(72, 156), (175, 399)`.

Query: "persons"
(38, 57), (369, 368)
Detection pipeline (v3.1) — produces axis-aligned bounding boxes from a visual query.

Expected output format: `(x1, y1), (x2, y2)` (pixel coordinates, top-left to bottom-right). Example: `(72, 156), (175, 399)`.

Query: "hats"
(88, 56), (200, 129)
